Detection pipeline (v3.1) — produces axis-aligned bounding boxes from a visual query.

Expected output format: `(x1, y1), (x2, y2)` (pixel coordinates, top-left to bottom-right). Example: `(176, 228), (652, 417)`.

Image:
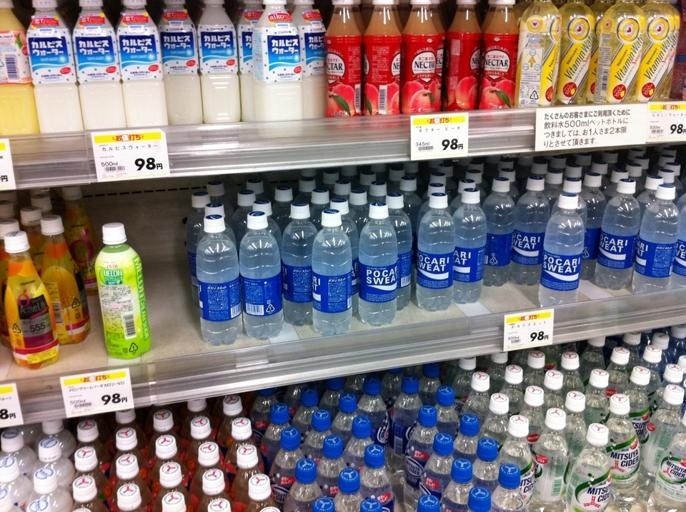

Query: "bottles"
(186, 140), (686, 347)
(0, 0), (686, 137)
(0, 185), (151, 370)
(0, 323), (686, 512)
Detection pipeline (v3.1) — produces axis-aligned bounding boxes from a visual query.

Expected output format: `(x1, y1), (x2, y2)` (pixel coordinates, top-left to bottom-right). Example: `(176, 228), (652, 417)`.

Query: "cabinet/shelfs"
(1, 101), (686, 432)
